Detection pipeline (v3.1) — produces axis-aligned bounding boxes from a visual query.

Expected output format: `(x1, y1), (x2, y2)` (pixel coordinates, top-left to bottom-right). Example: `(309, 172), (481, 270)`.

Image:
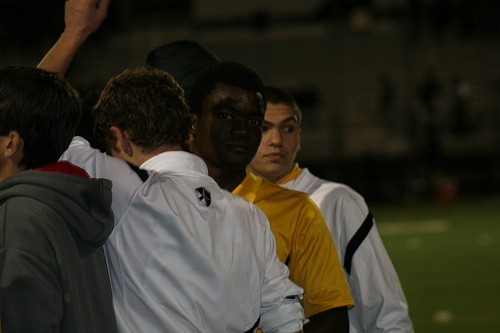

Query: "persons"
(0, 65), (117, 333)
(247, 87), (415, 333)
(186, 60), (357, 333)
(33, 1), (310, 333)
(145, 39), (220, 117)
